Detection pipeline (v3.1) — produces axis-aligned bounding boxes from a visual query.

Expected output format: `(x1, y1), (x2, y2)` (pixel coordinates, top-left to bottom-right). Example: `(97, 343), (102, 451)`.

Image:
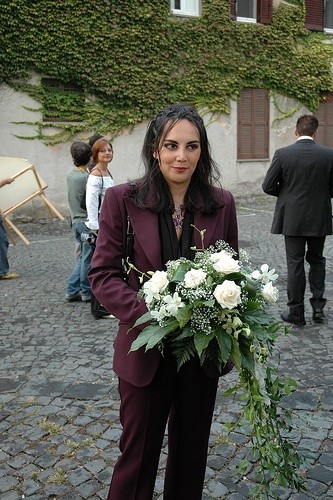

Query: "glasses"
(99, 149), (112, 152)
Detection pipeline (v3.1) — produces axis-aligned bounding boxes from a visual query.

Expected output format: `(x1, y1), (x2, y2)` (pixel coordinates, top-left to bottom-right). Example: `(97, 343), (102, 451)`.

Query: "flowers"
(121, 223), (317, 500)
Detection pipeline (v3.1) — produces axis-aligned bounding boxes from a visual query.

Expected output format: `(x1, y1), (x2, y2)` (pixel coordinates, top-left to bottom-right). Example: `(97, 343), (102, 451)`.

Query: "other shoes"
(1, 274), (20, 279)
(64, 295), (82, 303)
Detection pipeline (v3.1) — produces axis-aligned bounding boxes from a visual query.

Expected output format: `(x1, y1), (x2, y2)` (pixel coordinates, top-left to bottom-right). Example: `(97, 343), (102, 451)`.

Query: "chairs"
(0, 156), (65, 247)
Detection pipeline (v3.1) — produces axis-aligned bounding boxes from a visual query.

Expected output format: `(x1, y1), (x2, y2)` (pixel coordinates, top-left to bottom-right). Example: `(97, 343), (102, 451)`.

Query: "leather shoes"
(312, 307), (324, 321)
(281, 313), (306, 327)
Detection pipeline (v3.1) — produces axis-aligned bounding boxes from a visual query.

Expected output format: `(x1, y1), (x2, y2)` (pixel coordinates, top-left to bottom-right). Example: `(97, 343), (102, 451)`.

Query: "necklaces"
(171, 208), (184, 229)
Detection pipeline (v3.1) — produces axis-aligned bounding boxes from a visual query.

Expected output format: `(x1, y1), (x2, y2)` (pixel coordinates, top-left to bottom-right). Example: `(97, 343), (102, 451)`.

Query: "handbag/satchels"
(79, 168), (104, 212)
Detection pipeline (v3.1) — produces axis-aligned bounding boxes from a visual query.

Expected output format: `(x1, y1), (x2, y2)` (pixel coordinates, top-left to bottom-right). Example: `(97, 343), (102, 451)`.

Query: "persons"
(65, 134), (115, 319)
(262, 115), (333, 326)
(0, 177), (19, 280)
(87, 102), (239, 500)
